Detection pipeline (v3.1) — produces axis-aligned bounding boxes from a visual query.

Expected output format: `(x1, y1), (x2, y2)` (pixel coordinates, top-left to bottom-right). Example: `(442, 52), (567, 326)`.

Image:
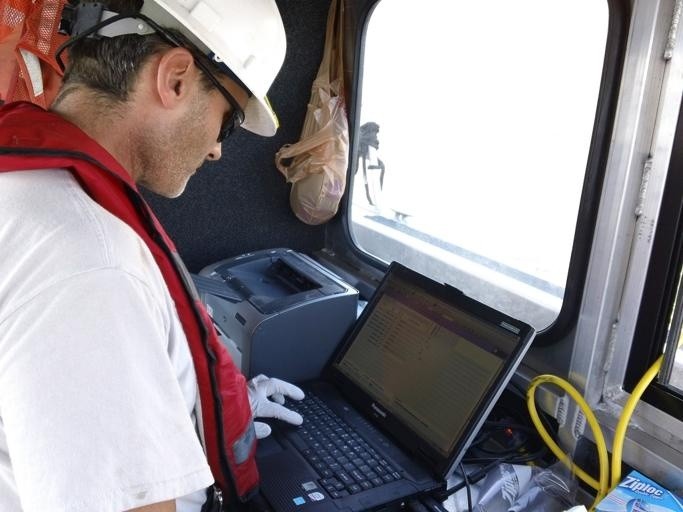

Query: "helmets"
(58, 0), (289, 138)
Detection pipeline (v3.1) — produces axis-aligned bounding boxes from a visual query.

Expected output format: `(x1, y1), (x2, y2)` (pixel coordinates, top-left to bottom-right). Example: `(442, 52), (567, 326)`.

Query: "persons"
(0, 1), (308, 512)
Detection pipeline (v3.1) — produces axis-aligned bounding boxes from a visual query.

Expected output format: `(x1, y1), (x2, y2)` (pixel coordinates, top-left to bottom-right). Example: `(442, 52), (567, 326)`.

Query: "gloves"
(245, 373), (305, 441)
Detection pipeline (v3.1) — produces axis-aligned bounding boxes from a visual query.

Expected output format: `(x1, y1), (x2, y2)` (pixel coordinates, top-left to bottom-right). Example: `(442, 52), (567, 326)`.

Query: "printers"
(187, 242), (361, 393)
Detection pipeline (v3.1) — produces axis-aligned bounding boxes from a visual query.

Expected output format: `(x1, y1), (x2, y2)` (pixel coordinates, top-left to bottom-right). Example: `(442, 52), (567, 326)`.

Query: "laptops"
(253, 259), (537, 511)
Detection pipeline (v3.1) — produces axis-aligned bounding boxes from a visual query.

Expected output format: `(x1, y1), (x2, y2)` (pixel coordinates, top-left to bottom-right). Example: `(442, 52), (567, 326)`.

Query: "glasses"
(197, 56), (246, 144)
